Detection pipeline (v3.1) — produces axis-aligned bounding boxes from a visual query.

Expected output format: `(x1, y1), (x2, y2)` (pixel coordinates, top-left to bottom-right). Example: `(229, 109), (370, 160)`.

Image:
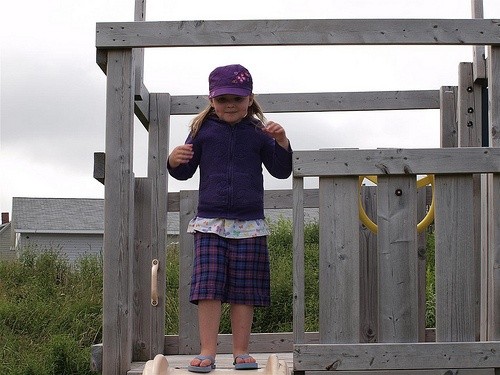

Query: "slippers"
(232, 354), (258, 370)
(188, 356), (216, 372)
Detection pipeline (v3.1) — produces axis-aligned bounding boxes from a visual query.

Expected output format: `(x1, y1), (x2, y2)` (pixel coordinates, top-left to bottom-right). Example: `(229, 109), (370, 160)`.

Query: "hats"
(208, 64), (253, 98)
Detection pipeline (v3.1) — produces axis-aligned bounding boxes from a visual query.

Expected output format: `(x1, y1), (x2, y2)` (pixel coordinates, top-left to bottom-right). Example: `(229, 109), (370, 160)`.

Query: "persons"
(166, 63), (291, 372)
